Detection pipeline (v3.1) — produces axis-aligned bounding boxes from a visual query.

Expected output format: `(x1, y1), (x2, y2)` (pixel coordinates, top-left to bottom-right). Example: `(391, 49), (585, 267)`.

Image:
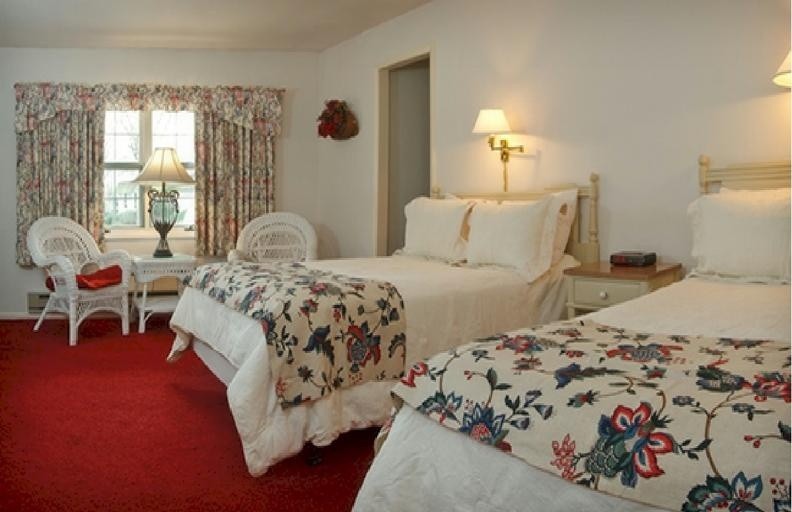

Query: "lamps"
(772, 50), (792, 88)
(131, 147), (198, 258)
(472, 108), (524, 192)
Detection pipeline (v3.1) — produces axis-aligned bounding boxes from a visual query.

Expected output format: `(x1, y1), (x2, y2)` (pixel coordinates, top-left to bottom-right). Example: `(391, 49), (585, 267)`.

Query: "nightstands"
(563, 260), (682, 320)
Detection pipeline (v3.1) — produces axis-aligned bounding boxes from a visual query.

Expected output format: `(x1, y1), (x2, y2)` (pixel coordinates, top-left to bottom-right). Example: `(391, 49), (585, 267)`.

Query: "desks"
(129, 254), (198, 334)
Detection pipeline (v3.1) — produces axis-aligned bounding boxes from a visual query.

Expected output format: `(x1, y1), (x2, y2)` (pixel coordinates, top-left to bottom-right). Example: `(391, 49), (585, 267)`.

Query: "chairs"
(227, 211), (319, 263)
(26, 216), (131, 346)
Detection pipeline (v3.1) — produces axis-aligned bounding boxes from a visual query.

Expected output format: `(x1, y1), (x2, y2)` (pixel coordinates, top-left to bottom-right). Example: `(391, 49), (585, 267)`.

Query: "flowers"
(317, 100), (347, 138)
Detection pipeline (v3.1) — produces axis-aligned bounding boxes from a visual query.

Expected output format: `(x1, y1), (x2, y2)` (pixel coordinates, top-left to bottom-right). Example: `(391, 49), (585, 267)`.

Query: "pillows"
(687, 188), (792, 286)
(402, 189), (578, 282)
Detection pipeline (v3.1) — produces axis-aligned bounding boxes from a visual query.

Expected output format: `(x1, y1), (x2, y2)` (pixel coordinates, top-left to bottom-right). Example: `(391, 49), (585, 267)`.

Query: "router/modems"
(610, 250), (656, 266)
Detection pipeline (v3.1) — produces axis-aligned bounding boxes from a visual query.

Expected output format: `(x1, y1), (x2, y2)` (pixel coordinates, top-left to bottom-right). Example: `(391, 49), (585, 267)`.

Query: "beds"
(411, 154), (792, 512)
(192, 173), (599, 465)
